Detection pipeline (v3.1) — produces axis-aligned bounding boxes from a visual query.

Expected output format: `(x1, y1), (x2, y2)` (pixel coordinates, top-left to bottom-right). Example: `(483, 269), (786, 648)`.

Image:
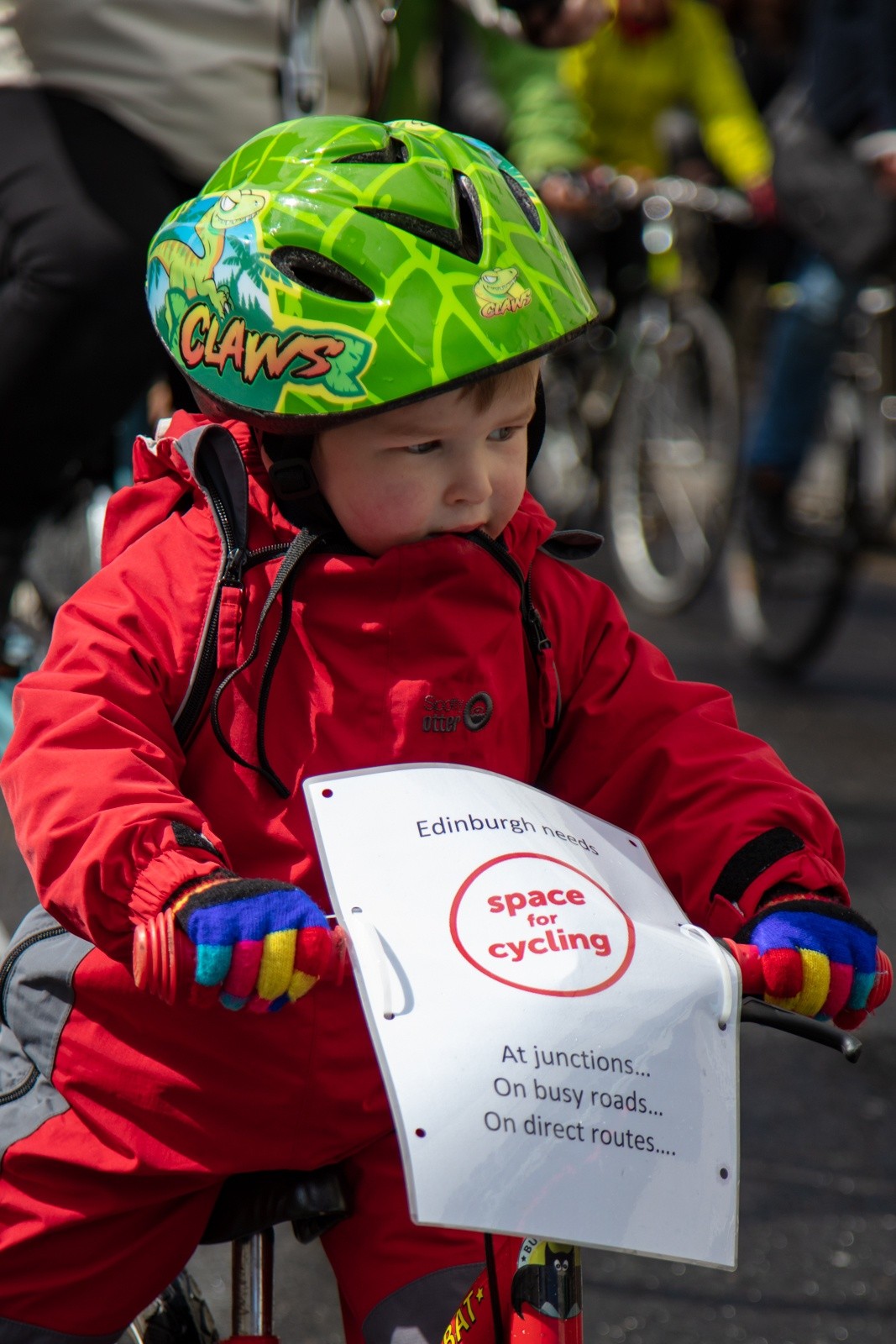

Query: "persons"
(0, 115), (880, 1344)
(0, 0), (896, 683)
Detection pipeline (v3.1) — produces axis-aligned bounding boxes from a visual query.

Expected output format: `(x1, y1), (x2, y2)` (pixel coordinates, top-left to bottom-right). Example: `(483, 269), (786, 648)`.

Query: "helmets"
(144, 114), (599, 439)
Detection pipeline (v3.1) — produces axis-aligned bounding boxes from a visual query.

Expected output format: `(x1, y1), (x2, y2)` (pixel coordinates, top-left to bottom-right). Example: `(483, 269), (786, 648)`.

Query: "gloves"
(752, 910), (879, 1030)
(172, 877), (333, 1015)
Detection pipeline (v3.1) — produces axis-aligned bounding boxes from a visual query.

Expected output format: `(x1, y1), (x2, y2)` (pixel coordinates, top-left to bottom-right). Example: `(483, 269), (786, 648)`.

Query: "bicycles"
(116, 905), (894, 1343)
(519, 159), (896, 684)
